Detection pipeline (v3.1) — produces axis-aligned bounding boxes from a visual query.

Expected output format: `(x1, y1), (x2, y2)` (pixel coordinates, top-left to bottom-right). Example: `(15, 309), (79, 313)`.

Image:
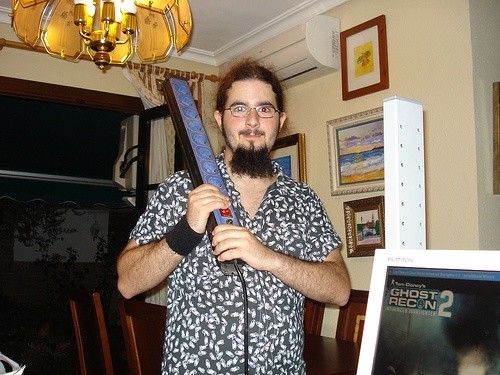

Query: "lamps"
(9, 0), (193, 71)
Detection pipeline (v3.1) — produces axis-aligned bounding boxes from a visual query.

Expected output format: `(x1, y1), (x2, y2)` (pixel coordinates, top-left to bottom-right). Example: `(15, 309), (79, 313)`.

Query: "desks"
(124, 299), (360, 375)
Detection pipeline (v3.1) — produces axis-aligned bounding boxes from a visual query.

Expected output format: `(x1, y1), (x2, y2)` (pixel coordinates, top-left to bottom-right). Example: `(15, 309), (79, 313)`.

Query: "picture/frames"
(271, 133), (307, 185)
(327, 106), (385, 195)
(343, 196), (385, 257)
(340, 14), (389, 101)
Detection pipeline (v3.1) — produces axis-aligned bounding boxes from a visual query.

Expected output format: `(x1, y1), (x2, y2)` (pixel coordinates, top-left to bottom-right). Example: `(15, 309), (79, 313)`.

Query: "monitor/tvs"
(357, 248), (500, 375)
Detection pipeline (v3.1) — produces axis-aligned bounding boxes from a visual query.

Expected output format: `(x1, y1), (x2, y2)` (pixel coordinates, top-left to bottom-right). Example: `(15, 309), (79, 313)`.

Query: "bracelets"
(166, 215), (204, 257)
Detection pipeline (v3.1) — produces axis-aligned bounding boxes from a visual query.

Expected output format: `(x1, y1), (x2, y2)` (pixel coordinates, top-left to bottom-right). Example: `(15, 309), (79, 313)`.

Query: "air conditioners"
(224, 15), (341, 91)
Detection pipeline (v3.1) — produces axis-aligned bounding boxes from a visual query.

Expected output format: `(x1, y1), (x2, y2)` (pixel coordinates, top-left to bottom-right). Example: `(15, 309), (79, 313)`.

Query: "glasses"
(222, 105), (281, 118)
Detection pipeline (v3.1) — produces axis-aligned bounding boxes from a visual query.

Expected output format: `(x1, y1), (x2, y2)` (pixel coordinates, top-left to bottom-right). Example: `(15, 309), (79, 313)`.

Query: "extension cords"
(160, 75), (246, 276)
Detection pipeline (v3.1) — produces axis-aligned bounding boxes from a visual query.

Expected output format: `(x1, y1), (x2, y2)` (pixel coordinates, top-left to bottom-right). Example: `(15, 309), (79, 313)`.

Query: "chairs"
(69, 289), (142, 375)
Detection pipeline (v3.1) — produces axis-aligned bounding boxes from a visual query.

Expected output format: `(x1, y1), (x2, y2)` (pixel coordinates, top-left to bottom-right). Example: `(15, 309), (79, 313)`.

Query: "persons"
(117, 61), (350, 375)
